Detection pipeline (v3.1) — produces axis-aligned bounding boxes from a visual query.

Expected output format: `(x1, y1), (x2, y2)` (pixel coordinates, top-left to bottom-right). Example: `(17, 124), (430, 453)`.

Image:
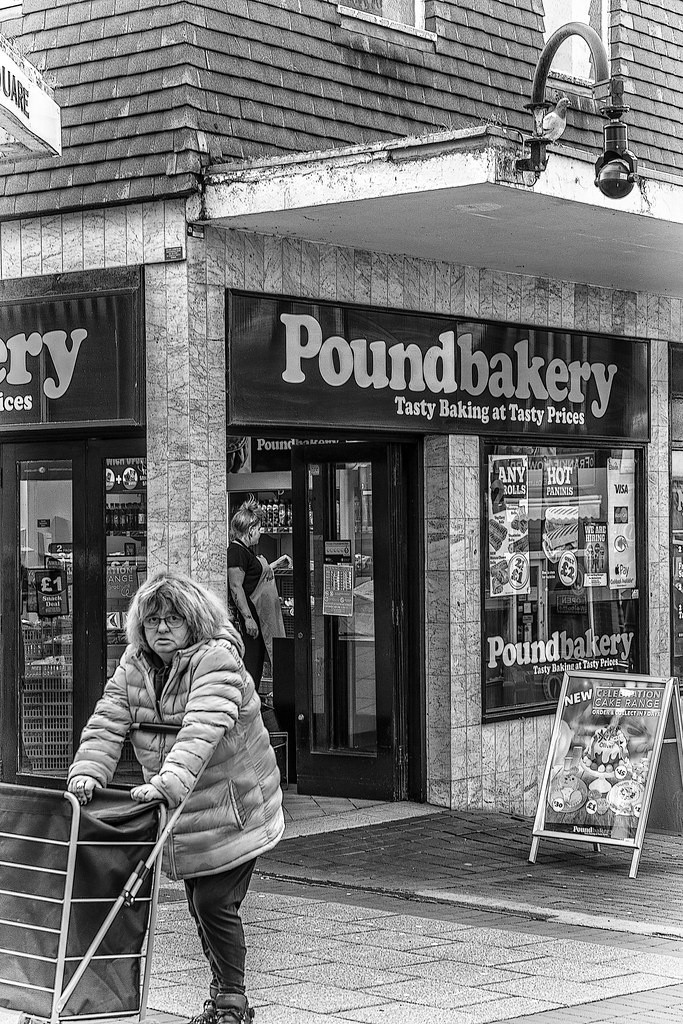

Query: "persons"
(67, 572), (286, 1024)
(227, 492), (288, 694)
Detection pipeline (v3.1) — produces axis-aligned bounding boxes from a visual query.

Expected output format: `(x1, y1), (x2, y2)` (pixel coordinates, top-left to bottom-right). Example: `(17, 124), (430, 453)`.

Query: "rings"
(77, 781), (84, 789)
(141, 791), (145, 794)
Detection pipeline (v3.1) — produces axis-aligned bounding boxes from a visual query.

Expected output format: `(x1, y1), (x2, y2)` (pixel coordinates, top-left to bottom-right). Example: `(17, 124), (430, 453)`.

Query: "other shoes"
(216, 993), (254, 1024)
(187, 999), (216, 1024)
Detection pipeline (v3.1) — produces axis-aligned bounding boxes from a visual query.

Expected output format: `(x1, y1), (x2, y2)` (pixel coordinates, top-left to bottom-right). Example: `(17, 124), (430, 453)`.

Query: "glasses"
(143, 615), (186, 629)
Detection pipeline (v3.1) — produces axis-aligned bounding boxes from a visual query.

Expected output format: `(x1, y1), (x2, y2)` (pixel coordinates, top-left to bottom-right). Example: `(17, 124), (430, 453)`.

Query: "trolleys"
(0, 722), (216, 1024)
